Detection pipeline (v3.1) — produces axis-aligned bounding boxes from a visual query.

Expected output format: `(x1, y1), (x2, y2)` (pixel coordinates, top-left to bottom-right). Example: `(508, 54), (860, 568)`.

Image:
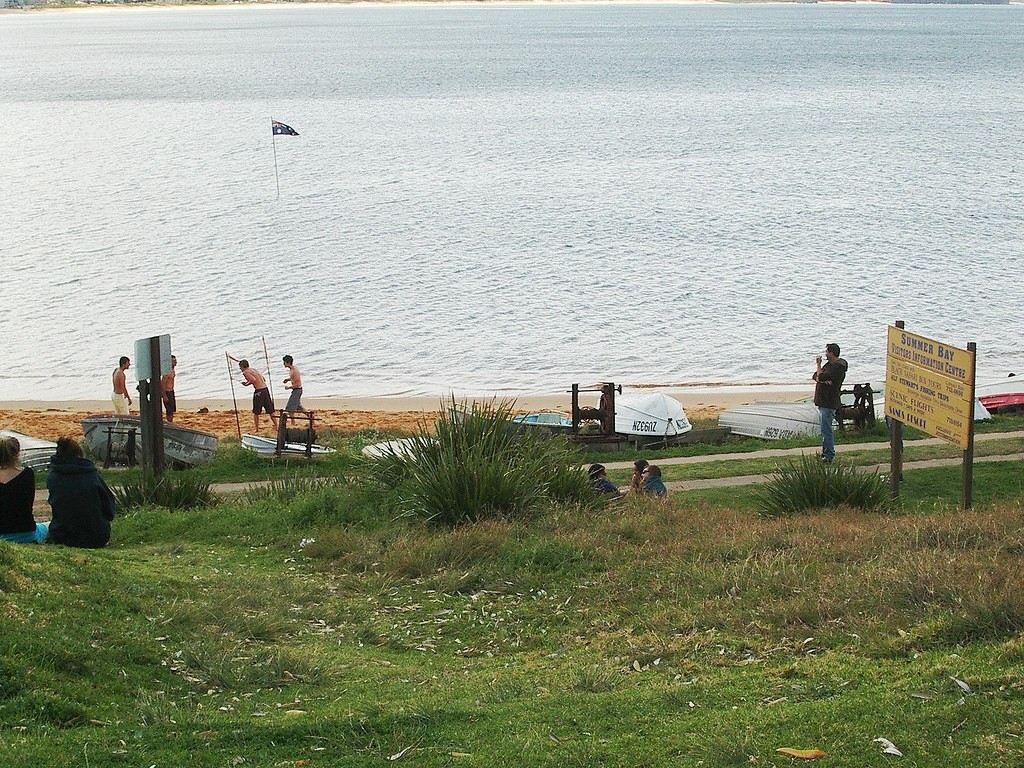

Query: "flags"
(272, 120), (300, 136)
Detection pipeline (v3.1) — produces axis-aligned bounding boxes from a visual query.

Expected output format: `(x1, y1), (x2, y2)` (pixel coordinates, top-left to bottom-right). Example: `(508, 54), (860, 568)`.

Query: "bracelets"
(163, 395), (166, 398)
(124, 393), (129, 399)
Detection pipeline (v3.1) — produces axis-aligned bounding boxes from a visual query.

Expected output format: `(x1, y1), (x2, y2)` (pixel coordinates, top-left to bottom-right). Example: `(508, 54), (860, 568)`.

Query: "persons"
(46, 437), (115, 549)
(239, 359), (278, 432)
(812, 343), (848, 463)
(161, 355), (177, 423)
(0, 436), (48, 544)
(111, 356), (132, 415)
(588, 458), (668, 499)
(283, 355), (314, 427)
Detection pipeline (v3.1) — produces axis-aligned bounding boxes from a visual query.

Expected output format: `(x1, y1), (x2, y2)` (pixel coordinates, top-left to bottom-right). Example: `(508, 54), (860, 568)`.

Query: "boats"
(363, 438), (441, 460)
(448, 402), (602, 432)
(718, 403), (834, 439)
(796, 371), (1024, 425)
(82, 412), (219, 467)
(0, 430), (60, 473)
(238, 433), (336, 457)
(592, 393), (693, 436)
